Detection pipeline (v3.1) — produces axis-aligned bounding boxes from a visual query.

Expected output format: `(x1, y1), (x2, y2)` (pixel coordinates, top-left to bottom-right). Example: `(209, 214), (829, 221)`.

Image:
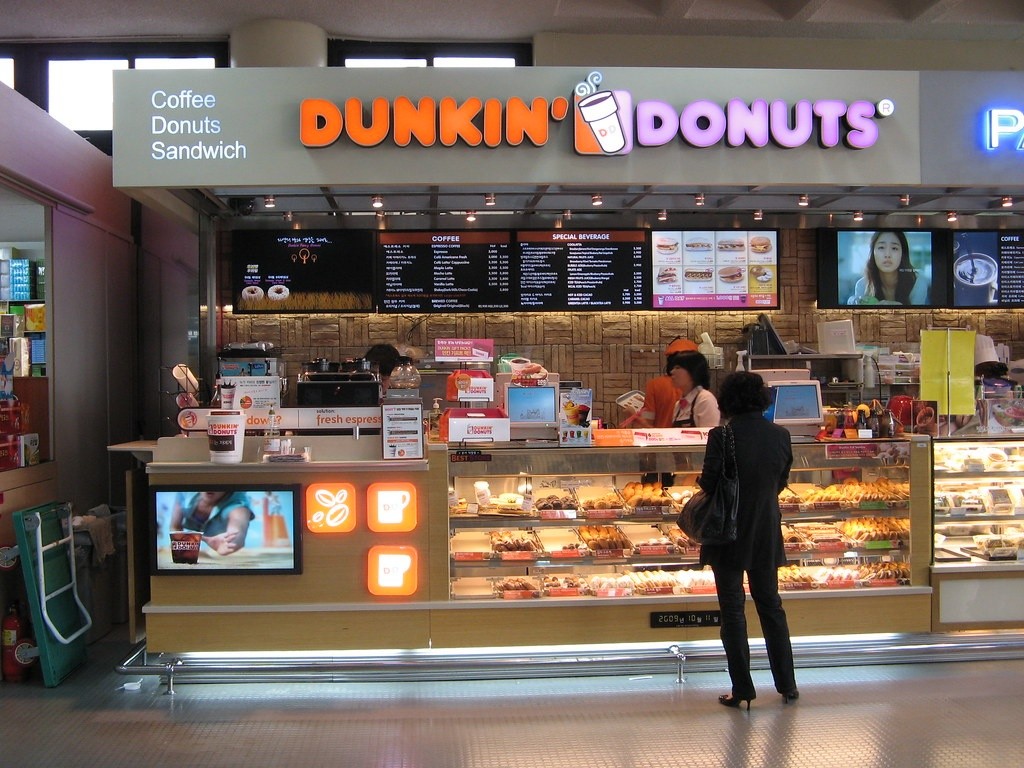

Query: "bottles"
(390, 356), (421, 390)
(211, 386), (222, 408)
(265, 405), (280, 457)
(827, 408), (896, 439)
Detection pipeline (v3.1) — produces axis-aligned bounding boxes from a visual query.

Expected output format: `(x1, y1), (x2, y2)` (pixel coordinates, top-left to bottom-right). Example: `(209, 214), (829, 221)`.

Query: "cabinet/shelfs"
(0, 300), (50, 462)
(448, 432), (934, 600)
(932, 433), (1024, 566)
(742, 331), (864, 429)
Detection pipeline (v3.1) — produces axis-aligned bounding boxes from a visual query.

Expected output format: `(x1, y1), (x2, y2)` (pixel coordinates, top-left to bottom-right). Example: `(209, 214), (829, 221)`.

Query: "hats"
(665, 339), (698, 355)
(973, 334), (999, 366)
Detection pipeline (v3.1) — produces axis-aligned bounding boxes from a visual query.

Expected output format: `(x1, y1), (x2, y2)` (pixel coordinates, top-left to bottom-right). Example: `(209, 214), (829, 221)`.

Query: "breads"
(929, 448), (1024, 555)
(448, 483), (714, 589)
(776, 480), (910, 582)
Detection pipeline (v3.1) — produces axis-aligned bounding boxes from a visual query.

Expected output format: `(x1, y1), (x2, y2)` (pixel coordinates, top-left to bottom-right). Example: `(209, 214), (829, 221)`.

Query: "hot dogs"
(717, 239), (746, 251)
(686, 241), (713, 251)
(684, 268), (713, 281)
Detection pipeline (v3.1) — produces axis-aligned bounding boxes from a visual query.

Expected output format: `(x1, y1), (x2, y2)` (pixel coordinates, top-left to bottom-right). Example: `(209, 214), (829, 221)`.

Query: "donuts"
(268, 284), (289, 301)
(242, 285), (264, 302)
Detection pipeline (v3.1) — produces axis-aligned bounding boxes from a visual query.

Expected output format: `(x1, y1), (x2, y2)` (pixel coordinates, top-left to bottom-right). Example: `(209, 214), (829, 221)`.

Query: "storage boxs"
(877, 363), (896, 370)
(9, 306), (25, 315)
(23, 304), (46, 332)
(438, 407), (511, 443)
(880, 370), (895, 377)
(16, 432), (39, 467)
(895, 371), (910, 377)
(895, 377), (912, 383)
(881, 378), (894, 383)
(448, 369), (494, 403)
(895, 364), (912, 371)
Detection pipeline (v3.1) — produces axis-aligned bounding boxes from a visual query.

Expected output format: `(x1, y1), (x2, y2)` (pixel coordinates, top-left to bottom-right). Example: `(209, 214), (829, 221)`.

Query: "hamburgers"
(718, 266), (744, 283)
(657, 266), (677, 284)
(750, 237), (772, 253)
(656, 238), (678, 254)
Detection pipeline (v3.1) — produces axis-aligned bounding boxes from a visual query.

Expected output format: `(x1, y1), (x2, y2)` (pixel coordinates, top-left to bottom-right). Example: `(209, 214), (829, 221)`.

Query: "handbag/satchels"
(676, 425), (739, 546)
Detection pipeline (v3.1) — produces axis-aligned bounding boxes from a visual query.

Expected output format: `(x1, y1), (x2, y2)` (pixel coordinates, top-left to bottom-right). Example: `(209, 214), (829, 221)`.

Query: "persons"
(692, 370), (800, 712)
(974, 335), (1018, 393)
(621, 338), (720, 486)
(847, 232), (927, 305)
(170, 492), (256, 555)
(362, 341), (402, 394)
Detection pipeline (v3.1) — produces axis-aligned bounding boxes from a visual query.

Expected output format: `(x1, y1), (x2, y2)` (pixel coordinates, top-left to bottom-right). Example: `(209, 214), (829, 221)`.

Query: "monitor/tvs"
(377, 228), (516, 314)
(815, 227), (951, 309)
(149, 483), (304, 576)
(768, 380), (824, 424)
(516, 227), (651, 312)
(503, 382), (559, 428)
(651, 227), (780, 311)
(952, 228), (1024, 309)
(231, 228), (376, 314)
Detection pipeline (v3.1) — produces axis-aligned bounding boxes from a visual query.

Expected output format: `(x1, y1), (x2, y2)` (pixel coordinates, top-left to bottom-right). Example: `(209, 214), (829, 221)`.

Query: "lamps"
(695, 193), (705, 206)
(283, 210), (293, 222)
(375, 211), (385, 222)
(562, 210), (571, 221)
(754, 209), (763, 221)
(264, 195), (275, 208)
(853, 210), (863, 221)
(371, 194), (384, 207)
(898, 195), (909, 206)
(484, 193), (496, 206)
(798, 194), (808, 206)
(592, 193), (602, 206)
(1002, 196), (1013, 207)
(465, 209), (477, 221)
(657, 209), (666, 220)
(947, 211), (957, 221)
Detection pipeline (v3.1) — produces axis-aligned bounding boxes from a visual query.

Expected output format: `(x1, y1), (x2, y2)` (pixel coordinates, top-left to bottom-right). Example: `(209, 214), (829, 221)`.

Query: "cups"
(509, 358), (530, 386)
(206, 414), (247, 461)
(916, 408), (936, 435)
(954, 253), (998, 304)
(169, 531), (204, 563)
(564, 405), (590, 425)
(222, 388), (236, 410)
(173, 364), (199, 408)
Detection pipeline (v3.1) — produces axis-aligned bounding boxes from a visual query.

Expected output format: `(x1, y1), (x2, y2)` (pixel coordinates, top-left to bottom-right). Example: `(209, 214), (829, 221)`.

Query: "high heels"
(781, 687), (799, 705)
(719, 691), (752, 711)
